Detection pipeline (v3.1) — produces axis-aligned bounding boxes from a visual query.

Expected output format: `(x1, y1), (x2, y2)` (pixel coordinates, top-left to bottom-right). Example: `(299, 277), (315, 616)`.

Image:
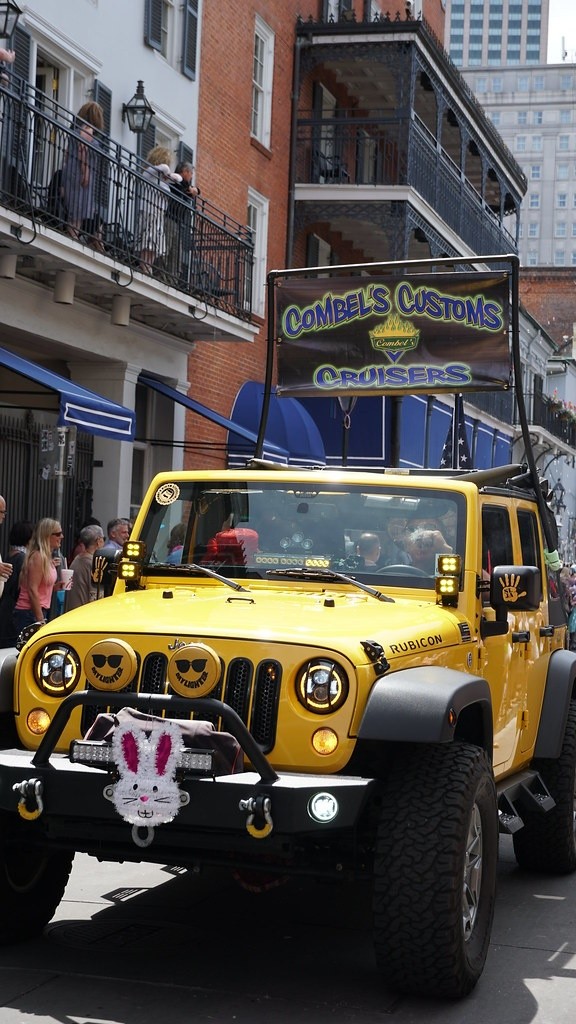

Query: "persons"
(0, 45), (16, 86)
(14, 518), (74, 636)
(93, 518), (129, 556)
(0, 495), (13, 599)
(131, 146), (183, 275)
(48, 171), (105, 251)
(163, 160), (201, 290)
(64, 525), (107, 613)
(344, 514), (490, 600)
(61, 101), (103, 236)
(560, 565), (576, 650)
(72, 517), (102, 558)
(0, 520), (61, 649)
(165, 524), (187, 565)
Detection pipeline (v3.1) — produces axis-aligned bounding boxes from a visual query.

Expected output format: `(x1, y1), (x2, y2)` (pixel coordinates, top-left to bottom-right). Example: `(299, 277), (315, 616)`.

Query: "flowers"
(547, 386), (576, 423)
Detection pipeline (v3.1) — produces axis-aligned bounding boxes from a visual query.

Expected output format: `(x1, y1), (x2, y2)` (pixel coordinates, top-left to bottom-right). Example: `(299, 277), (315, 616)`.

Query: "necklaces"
(87, 551), (93, 555)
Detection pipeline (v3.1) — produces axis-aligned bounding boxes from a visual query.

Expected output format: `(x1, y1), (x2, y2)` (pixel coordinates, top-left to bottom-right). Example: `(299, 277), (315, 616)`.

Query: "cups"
(1, 562), (13, 583)
(60, 568), (74, 590)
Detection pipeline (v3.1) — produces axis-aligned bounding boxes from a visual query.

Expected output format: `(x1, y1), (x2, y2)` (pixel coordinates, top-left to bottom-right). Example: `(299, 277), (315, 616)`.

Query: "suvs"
(1, 467), (576, 1009)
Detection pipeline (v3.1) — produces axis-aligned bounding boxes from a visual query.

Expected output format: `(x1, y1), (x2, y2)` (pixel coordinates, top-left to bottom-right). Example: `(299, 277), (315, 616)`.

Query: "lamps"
(122, 79), (156, 133)
(0, 0), (24, 39)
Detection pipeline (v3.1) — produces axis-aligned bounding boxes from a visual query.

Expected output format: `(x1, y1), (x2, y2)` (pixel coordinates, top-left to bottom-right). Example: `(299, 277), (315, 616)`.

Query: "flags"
(440, 394), (473, 469)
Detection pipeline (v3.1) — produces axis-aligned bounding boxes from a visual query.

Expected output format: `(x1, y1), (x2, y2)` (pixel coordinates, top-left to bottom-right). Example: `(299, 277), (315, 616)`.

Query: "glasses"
(51, 532), (61, 537)
(0, 511), (8, 515)
(402, 523), (441, 534)
(95, 536), (106, 542)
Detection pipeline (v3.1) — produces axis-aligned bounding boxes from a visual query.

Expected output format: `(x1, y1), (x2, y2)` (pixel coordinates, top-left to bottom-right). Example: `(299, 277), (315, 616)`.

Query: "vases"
(560, 414), (568, 420)
(548, 405), (557, 413)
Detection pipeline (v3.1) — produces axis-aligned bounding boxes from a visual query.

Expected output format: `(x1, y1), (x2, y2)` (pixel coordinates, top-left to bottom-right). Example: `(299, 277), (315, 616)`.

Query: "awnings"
(0, 350), (135, 443)
(227, 381), (326, 469)
(136, 374), (290, 465)
